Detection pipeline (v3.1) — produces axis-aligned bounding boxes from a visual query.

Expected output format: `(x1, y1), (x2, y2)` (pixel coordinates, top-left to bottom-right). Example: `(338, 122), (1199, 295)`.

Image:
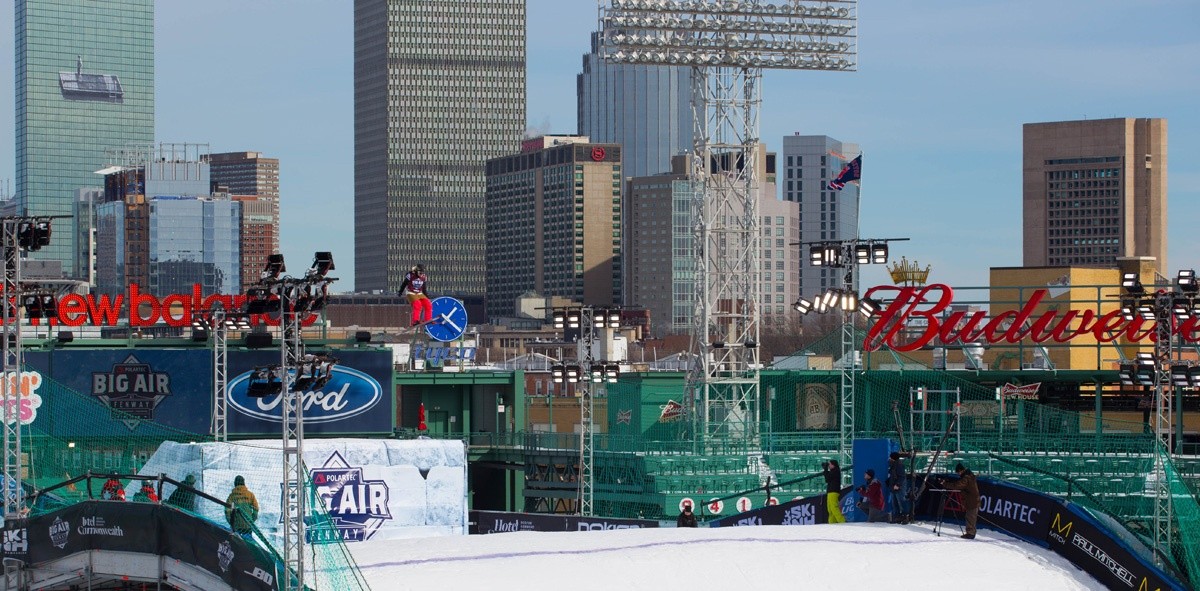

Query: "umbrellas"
(418, 401), (427, 437)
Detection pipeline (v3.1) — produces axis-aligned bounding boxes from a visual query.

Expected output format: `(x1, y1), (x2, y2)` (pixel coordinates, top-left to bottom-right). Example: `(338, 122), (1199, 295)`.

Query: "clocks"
(425, 296), (467, 343)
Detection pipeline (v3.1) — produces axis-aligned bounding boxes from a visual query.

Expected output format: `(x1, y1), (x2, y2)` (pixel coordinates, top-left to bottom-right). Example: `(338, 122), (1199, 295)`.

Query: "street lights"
(783, 235), (917, 490)
(597, 1), (859, 480)
(247, 251), (343, 589)
(1110, 268), (1200, 573)
(0, 213), (56, 525)
(536, 303), (628, 525)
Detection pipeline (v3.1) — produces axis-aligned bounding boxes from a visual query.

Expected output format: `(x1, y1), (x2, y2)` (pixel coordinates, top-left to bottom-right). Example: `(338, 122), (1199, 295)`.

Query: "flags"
(827, 154), (862, 192)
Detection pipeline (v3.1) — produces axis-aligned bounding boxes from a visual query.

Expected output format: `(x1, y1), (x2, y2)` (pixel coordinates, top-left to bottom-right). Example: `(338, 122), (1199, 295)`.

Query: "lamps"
(58, 332), (74, 343)
(794, 287), (880, 318)
(263, 254), (286, 278)
(356, 331), (372, 344)
(0, 217), (52, 253)
(312, 251), (335, 276)
(809, 240), (887, 265)
(246, 332), (273, 347)
(712, 342), (724, 348)
(744, 341), (756, 348)
(25, 294), (59, 318)
(1119, 352), (1200, 387)
(553, 305), (622, 328)
(552, 361), (620, 383)
(1121, 271), (1200, 315)
(247, 361), (339, 396)
(246, 284), (330, 313)
(192, 329), (208, 342)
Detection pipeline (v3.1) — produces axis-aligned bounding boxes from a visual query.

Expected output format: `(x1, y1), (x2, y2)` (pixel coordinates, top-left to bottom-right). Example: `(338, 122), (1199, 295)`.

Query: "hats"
(185, 475), (196, 485)
(234, 476), (245, 487)
(955, 463), (964, 473)
(830, 460), (838, 467)
(414, 264), (425, 274)
(685, 506), (691, 512)
(889, 452), (900, 461)
(866, 469), (875, 478)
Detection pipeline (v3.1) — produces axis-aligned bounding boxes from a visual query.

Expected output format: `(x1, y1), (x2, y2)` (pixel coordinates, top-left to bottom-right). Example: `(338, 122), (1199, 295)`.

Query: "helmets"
(141, 480), (153, 487)
(110, 472), (118, 481)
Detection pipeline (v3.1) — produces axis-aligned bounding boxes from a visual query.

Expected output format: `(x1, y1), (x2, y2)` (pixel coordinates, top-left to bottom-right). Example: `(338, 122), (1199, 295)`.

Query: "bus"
(0, 279), (90, 327)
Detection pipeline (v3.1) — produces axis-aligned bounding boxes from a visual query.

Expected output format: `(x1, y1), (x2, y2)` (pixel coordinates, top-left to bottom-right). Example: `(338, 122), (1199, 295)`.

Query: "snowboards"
(394, 315), (441, 336)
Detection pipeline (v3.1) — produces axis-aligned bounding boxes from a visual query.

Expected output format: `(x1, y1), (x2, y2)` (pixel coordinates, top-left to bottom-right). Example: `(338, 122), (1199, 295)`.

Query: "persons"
(224, 475), (259, 540)
(168, 474), (198, 512)
(938, 462), (980, 540)
(134, 480), (157, 503)
(859, 467), (885, 522)
(397, 263), (436, 329)
(823, 459), (847, 524)
(101, 470), (127, 501)
(887, 451), (910, 525)
(676, 506), (699, 528)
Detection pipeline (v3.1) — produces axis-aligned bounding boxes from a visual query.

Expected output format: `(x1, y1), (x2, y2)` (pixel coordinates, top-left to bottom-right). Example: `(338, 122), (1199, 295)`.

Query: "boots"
(890, 515), (901, 523)
(902, 513), (908, 525)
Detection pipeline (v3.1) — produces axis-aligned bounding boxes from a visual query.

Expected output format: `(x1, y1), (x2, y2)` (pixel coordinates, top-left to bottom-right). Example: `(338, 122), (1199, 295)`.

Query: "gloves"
(821, 462), (829, 470)
(397, 293), (402, 297)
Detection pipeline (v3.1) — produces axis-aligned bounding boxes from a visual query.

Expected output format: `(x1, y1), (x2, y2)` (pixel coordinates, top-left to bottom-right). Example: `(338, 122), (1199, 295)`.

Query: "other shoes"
(413, 321), (421, 326)
(422, 321), (437, 324)
(961, 533), (975, 539)
